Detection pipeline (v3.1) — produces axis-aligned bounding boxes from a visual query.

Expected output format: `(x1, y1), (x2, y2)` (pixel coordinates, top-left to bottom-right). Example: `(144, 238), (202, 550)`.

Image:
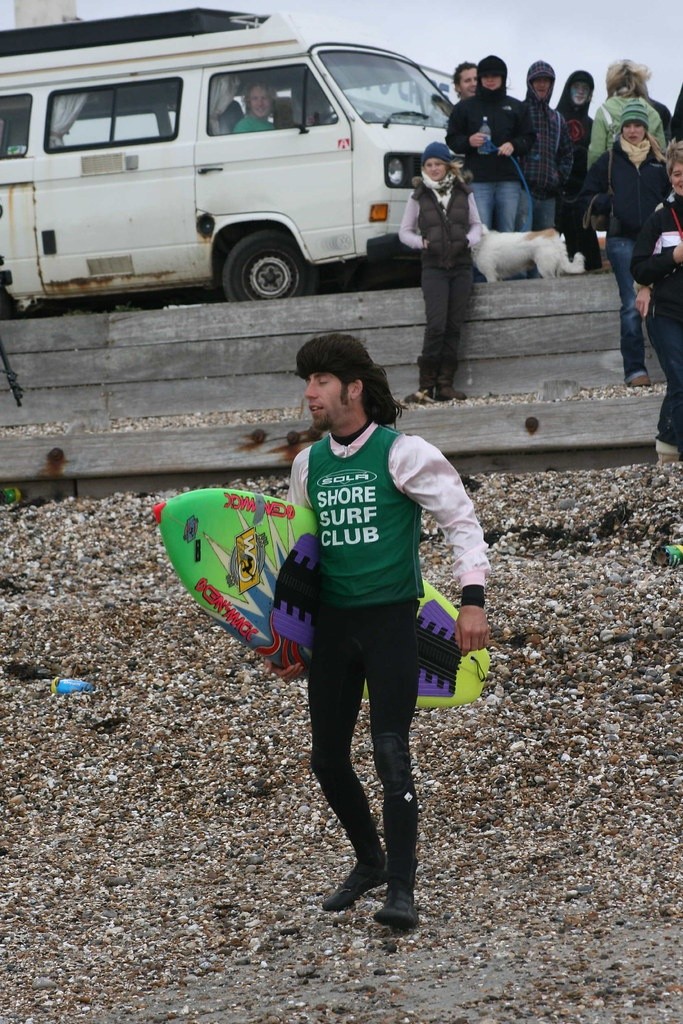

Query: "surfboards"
(156, 488), (489, 707)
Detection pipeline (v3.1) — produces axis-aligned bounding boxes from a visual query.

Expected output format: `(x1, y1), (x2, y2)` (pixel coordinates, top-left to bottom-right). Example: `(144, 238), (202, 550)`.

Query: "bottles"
(51, 677), (95, 693)
(478, 116), (491, 155)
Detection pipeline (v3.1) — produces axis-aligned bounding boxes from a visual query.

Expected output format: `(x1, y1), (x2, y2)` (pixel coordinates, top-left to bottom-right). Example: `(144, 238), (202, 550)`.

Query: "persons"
(445, 54), (536, 283)
(399, 142), (484, 403)
(231, 75), (277, 135)
(430, 94), (453, 116)
(555, 71), (607, 277)
(452, 60), (478, 99)
(587, 59), (683, 464)
(519, 59), (573, 281)
(262, 331), (492, 930)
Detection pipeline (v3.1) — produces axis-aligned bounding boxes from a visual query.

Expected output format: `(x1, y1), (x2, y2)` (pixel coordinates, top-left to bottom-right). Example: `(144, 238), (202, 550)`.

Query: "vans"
(0, 29), (456, 313)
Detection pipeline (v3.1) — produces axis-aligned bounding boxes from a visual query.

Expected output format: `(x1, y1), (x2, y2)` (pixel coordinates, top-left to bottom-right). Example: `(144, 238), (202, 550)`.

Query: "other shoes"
(376, 887), (418, 926)
(631, 375), (651, 386)
(323, 852), (389, 910)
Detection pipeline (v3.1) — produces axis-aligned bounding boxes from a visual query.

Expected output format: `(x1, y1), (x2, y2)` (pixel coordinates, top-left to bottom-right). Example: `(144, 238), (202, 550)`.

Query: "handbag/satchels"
(582, 149), (614, 230)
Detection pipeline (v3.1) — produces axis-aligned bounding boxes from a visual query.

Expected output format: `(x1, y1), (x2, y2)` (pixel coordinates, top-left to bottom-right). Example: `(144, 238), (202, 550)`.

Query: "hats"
(422, 142), (450, 168)
(530, 73), (555, 79)
(620, 101), (648, 130)
(477, 55), (506, 77)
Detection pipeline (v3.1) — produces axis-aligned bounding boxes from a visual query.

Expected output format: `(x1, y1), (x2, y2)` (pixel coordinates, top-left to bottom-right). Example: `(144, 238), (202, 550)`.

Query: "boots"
(435, 360), (466, 402)
(404, 356), (438, 403)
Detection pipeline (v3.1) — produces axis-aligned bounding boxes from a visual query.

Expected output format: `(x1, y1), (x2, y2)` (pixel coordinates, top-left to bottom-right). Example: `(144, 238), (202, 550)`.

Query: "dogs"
(470, 223), (586, 283)
